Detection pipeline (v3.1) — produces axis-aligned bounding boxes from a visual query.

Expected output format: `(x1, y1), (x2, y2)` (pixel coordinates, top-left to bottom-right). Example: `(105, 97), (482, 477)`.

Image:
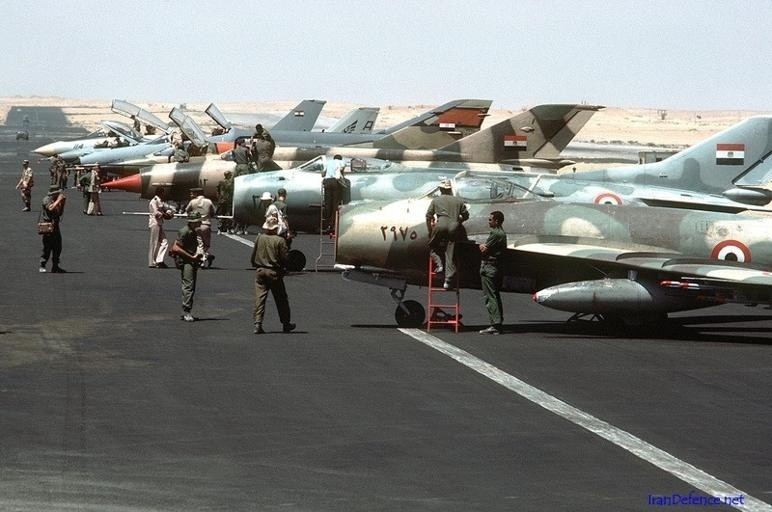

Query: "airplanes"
(31, 98), (327, 157)
(230, 114), (772, 233)
(333, 176), (772, 337)
(100, 103), (608, 203)
(99, 98), (495, 176)
(38, 107), (381, 164)
(70, 99), (469, 167)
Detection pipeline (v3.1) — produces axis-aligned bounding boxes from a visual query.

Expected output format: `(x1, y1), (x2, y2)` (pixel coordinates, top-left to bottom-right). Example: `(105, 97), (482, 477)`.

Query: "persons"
(251, 217), (297, 334)
(217, 171), (233, 236)
(186, 187), (217, 268)
(115, 136), (122, 147)
(260, 192), (278, 219)
(274, 189), (290, 228)
(38, 185), (67, 273)
(481, 211), (508, 334)
(425, 179), (470, 289)
(15, 160), (34, 212)
(149, 187), (176, 268)
(173, 211), (202, 322)
(322, 155), (347, 232)
(280, 229), (297, 248)
(80, 168), (93, 215)
(182, 189), (196, 212)
(233, 222), (248, 235)
(130, 115), (140, 131)
(256, 123), (274, 144)
(256, 135), (282, 171)
(233, 138), (251, 176)
(49, 153), (69, 189)
(174, 145), (189, 163)
(170, 131), (182, 144)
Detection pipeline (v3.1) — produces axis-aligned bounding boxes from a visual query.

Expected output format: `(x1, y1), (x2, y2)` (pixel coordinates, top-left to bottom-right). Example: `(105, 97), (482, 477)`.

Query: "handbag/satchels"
(36, 211), (54, 234)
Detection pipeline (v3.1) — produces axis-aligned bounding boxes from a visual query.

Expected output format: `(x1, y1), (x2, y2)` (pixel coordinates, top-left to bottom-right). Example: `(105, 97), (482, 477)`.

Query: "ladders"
(315, 182), (343, 272)
(428, 219), (461, 333)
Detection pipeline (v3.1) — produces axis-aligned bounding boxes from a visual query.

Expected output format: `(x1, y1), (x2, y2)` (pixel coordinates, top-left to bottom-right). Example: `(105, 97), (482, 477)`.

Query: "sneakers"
(443, 282), (452, 289)
(434, 267), (444, 273)
(479, 326), (500, 335)
(283, 322), (296, 331)
(183, 314), (195, 322)
(254, 326), (264, 334)
(39, 267), (46, 273)
(51, 266), (65, 273)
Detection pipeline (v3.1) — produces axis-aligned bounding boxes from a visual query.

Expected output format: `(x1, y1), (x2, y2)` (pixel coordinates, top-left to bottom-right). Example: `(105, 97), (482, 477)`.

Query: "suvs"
(15, 129), (29, 140)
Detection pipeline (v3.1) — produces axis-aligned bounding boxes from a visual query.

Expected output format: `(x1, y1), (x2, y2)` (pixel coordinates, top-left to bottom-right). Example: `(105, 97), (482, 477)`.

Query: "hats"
(437, 180), (452, 189)
(187, 212), (204, 222)
(260, 191), (274, 201)
(22, 160), (29, 165)
(262, 216), (279, 230)
(47, 185), (62, 195)
